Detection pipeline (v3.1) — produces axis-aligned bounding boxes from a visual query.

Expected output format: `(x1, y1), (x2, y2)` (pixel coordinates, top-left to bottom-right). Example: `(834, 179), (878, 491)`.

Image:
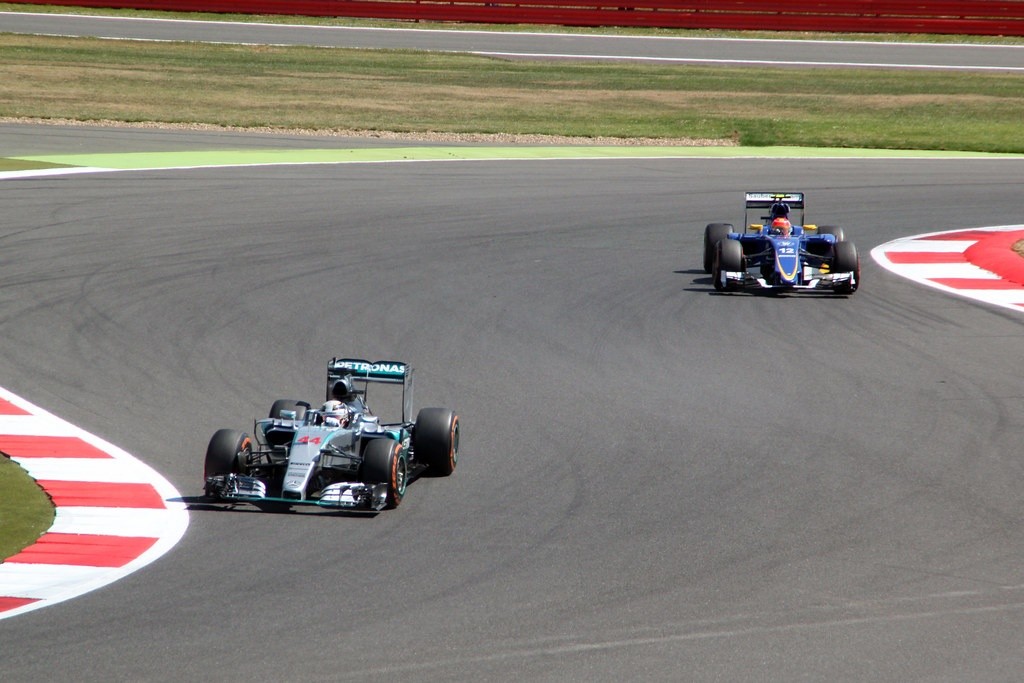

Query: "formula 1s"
(200, 355), (461, 514)
(701, 187), (861, 297)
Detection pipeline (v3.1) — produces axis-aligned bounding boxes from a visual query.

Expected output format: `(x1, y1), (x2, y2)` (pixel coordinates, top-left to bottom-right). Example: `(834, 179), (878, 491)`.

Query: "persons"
(767, 217), (791, 238)
(314, 399), (349, 429)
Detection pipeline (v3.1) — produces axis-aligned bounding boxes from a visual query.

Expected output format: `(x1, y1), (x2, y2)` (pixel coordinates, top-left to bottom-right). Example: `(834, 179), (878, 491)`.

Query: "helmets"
(770, 217), (793, 236)
(318, 400), (350, 427)
